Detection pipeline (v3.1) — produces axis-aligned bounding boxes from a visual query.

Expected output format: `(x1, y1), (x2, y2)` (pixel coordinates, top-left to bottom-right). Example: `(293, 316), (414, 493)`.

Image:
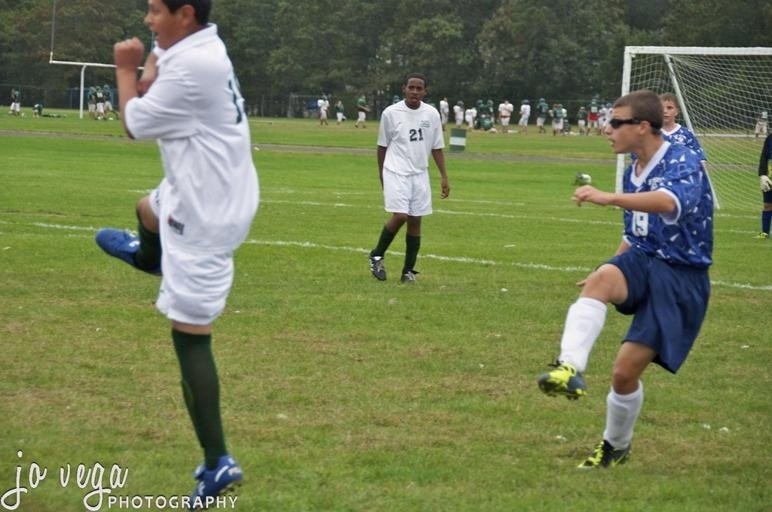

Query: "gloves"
(759, 175), (772, 193)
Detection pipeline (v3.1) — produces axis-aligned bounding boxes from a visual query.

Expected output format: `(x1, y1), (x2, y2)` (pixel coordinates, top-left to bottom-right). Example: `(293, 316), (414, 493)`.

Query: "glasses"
(610, 118), (639, 129)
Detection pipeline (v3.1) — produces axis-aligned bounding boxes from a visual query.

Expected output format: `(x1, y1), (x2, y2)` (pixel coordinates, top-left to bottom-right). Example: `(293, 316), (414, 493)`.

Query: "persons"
(95, 1), (263, 512)
(32, 104), (43, 119)
(426, 94), (613, 139)
(367, 73), (450, 283)
(86, 84), (120, 121)
(10, 88), (21, 117)
(536, 90), (713, 471)
(316, 95), (330, 127)
(334, 96), (348, 124)
(354, 92), (370, 128)
(752, 137), (772, 240)
(753, 107), (770, 142)
(629, 90), (707, 170)
(392, 94), (401, 103)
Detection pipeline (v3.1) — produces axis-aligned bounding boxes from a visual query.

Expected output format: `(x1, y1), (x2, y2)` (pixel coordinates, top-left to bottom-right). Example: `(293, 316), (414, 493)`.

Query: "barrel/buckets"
(449, 128), (467, 152)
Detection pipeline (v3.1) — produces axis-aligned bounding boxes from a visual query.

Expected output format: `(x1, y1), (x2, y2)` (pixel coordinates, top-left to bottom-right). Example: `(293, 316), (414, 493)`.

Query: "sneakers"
(401, 272), (424, 292)
(187, 456), (242, 510)
(95, 228), (162, 276)
(578, 438), (630, 469)
(369, 250), (387, 281)
(539, 360), (586, 401)
